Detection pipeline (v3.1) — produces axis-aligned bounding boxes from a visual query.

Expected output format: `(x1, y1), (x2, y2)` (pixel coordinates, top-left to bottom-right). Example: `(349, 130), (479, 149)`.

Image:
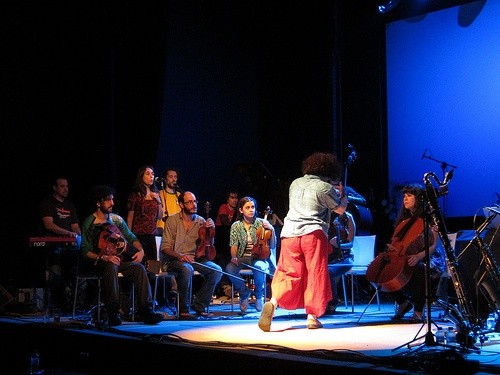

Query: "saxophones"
(427, 169), (478, 335)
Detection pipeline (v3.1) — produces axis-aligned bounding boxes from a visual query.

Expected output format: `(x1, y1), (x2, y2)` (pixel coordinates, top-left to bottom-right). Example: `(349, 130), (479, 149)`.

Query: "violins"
(251, 206), (272, 260)
(330, 217), (348, 260)
(195, 201), (217, 263)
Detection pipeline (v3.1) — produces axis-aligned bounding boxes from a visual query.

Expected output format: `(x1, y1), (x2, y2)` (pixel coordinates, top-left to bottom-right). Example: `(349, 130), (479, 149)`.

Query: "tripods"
(388, 190), (467, 354)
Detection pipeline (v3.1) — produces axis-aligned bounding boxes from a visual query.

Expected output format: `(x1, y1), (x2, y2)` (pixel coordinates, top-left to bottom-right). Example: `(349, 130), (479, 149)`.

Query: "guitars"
(95, 223), (161, 274)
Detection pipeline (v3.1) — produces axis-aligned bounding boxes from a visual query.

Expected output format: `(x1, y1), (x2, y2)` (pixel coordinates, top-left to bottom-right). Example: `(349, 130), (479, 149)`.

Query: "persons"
(258, 151), (348, 329)
(329, 176), (374, 235)
(325, 212), (356, 314)
(225, 196), (277, 310)
(160, 191), (223, 316)
(263, 203), (284, 226)
(41, 175), (83, 315)
(216, 192), (242, 226)
(126, 165), (164, 304)
(390, 183), (437, 321)
(156, 169), (184, 230)
(80, 186), (163, 327)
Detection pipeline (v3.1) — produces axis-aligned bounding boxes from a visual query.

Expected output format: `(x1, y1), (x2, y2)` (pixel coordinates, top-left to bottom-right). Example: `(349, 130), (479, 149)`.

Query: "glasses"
(184, 200), (198, 205)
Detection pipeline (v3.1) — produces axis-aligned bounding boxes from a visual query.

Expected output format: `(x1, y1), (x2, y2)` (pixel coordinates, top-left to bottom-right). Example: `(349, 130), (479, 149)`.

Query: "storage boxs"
(18, 288), (43, 310)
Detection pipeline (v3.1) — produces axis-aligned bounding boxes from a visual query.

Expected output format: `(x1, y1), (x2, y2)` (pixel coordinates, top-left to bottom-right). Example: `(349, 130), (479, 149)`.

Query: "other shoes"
(256, 296), (264, 312)
(307, 319), (323, 328)
(190, 303), (208, 316)
(411, 310), (424, 321)
(179, 311), (190, 318)
(326, 302), (337, 315)
(240, 292), (251, 311)
(258, 302), (274, 332)
(142, 311), (163, 325)
(393, 302), (414, 319)
(107, 312), (121, 325)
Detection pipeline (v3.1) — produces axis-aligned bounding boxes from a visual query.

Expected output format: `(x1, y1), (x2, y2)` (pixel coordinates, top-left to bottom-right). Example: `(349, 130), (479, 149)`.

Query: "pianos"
(29, 236), (77, 309)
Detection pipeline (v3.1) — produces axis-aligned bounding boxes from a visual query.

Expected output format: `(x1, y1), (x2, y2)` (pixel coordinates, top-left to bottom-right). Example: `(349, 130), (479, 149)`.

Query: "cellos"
(355, 184), (449, 322)
(344, 144), (373, 236)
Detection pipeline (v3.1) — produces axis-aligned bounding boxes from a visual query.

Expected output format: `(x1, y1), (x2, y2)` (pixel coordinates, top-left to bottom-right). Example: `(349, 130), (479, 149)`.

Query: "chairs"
(231, 247), (276, 313)
(72, 235), (136, 322)
(341, 234), (381, 314)
(437, 232), (458, 278)
(152, 235), (210, 318)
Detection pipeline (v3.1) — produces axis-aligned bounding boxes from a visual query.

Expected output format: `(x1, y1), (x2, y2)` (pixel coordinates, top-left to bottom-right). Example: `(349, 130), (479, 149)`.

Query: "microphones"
(154, 176), (163, 182)
(171, 182), (180, 188)
(487, 208), (500, 215)
